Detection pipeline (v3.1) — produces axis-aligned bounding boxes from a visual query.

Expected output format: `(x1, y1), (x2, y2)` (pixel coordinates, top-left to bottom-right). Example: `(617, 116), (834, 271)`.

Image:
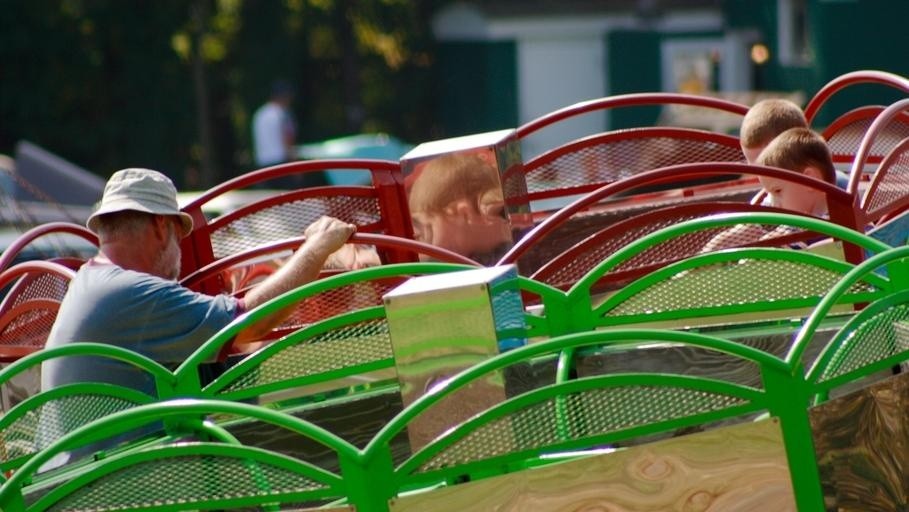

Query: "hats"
(86, 167), (194, 237)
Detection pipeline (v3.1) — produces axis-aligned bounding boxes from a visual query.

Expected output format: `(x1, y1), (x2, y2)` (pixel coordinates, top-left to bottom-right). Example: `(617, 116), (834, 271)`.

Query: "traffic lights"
(746, 39), (774, 68)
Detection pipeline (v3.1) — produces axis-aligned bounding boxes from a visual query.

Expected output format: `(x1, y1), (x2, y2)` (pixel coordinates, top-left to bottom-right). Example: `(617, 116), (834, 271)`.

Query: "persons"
(753, 126), (847, 253)
(248, 76), (297, 188)
(410, 157), (514, 273)
(36, 164), (357, 472)
(685, 98), (850, 268)
